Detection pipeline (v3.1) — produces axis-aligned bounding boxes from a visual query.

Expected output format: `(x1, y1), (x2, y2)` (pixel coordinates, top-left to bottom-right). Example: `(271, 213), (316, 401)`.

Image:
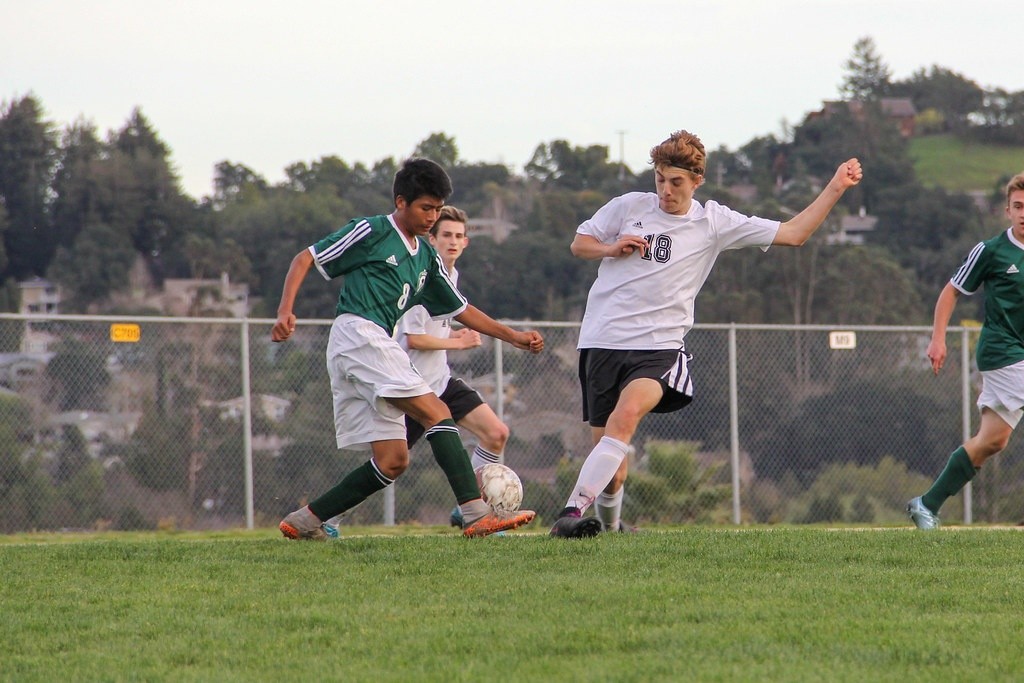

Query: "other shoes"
(320, 521), (338, 538)
(449, 508), (463, 529)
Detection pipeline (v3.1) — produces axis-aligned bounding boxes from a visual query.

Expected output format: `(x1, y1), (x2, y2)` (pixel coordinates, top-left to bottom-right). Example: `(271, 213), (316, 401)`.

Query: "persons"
(320, 206), (510, 538)
(906, 174), (1024, 528)
(271, 157), (547, 540)
(550, 131), (863, 537)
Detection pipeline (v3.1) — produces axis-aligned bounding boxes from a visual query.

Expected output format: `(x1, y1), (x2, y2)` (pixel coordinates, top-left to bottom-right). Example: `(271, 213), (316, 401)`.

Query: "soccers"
(457, 463), (523, 515)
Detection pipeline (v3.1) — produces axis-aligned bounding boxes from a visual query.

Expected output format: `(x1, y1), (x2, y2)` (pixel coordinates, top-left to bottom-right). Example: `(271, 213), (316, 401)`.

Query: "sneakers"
(550, 506), (601, 537)
(460, 503), (536, 539)
(619, 522), (638, 533)
(907, 494), (939, 530)
(278, 512), (327, 540)
